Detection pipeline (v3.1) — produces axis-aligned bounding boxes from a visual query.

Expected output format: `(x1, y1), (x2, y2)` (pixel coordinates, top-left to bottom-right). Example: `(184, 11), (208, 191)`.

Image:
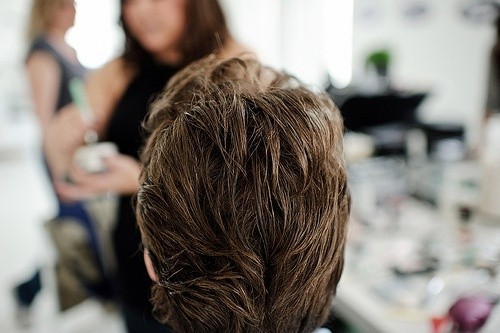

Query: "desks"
(326, 154), (499, 333)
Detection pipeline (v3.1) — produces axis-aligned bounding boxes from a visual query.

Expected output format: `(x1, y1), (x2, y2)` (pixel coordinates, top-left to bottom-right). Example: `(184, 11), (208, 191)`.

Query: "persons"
(44, 1), (235, 332)
(19, 1), (100, 295)
(133, 45), (352, 333)
